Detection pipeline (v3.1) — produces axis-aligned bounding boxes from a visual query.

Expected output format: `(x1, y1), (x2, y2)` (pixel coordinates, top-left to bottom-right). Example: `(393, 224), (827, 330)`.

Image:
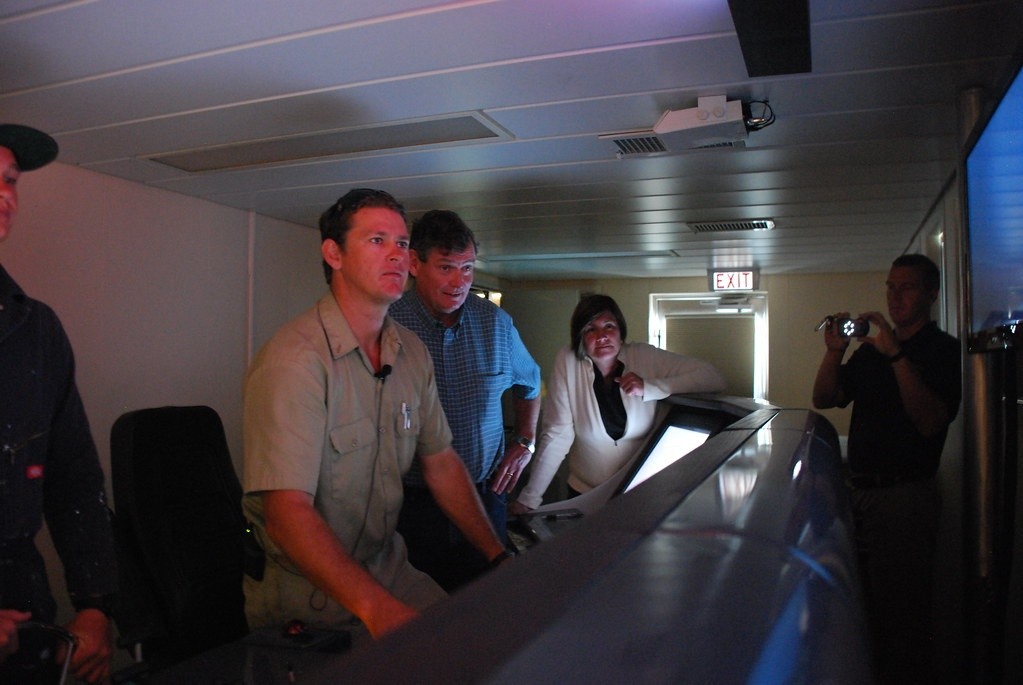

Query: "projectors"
(655, 100), (752, 155)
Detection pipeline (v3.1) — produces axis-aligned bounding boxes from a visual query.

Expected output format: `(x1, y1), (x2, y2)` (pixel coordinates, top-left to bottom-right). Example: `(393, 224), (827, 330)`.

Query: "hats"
(0, 124), (60, 171)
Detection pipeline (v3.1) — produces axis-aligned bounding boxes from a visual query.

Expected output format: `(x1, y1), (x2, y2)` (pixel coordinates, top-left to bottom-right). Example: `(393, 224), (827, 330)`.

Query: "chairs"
(110, 405), (249, 665)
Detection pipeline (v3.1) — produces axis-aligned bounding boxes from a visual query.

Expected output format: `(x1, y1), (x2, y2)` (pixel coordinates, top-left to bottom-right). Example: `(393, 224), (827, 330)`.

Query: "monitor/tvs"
(608, 406), (732, 503)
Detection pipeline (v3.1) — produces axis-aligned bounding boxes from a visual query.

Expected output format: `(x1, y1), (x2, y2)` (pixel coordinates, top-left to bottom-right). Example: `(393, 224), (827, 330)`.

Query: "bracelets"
(488, 549), (516, 568)
(888, 348), (908, 365)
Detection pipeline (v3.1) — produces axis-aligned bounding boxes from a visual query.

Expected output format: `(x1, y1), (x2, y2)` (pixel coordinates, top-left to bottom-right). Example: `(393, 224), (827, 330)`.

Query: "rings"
(506, 473), (514, 476)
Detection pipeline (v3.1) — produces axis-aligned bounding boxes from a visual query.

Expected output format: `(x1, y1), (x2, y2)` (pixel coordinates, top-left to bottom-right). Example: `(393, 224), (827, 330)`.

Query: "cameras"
(838, 318), (870, 337)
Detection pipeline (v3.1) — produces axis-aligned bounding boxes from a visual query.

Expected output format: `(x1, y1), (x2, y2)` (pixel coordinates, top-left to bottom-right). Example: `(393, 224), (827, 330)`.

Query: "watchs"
(513, 434), (536, 455)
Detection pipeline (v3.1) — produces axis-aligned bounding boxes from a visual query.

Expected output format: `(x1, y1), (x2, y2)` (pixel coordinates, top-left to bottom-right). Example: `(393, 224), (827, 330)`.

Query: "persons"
(512, 294), (728, 518)
(811, 253), (963, 684)
(385, 210), (544, 598)
(0, 120), (132, 685)
(238, 184), (520, 659)
